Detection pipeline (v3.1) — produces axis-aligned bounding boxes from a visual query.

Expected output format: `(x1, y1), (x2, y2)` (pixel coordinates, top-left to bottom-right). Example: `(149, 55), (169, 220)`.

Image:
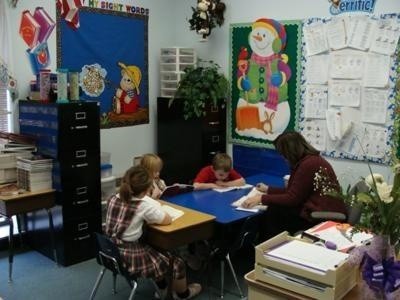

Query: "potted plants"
(168, 57), (229, 121)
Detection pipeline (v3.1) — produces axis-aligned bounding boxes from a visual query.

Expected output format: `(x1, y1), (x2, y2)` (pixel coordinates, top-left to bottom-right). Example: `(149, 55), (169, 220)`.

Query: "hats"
(116, 61), (143, 96)
(252, 18), (288, 48)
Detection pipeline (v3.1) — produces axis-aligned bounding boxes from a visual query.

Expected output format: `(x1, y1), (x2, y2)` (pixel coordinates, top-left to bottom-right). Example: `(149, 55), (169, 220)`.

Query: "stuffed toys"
(196, 0), (212, 35)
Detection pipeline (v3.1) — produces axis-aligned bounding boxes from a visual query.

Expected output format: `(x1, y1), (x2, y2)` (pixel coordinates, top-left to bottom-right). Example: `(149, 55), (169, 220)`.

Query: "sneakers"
(154, 282), (202, 300)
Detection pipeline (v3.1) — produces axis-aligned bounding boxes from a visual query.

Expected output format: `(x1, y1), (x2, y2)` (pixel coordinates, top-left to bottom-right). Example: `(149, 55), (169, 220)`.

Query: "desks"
(0, 189), (58, 271)
(141, 171), (285, 282)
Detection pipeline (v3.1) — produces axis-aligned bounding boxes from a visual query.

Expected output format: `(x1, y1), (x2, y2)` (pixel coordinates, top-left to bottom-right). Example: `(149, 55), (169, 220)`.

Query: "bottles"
(38, 67), (82, 104)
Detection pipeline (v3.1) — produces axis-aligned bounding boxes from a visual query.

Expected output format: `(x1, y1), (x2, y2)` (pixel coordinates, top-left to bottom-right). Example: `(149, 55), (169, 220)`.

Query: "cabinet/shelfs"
(18, 99), (101, 267)
(155, 95), (226, 186)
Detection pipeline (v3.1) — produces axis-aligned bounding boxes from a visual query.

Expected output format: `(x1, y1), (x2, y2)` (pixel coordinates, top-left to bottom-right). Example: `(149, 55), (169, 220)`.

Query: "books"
(19, 10), (40, 48)
(35, 7), (55, 43)
(15, 151), (54, 193)
(230, 186), (268, 213)
(26, 44), (51, 74)
(159, 184), (194, 199)
(262, 220), (372, 292)
(0, 183), (27, 196)
(213, 183), (254, 193)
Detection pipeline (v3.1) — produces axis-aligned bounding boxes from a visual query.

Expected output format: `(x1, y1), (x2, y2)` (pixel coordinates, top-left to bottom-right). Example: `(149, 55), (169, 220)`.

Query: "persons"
(193, 153), (246, 240)
(140, 152), (202, 271)
(235, 131), (348, 266)
(104, 164), (203, 300)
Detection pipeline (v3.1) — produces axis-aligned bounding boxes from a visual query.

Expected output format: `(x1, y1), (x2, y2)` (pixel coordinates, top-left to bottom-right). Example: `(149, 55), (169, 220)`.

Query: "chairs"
(89, 233), (163, 300)
(311, 177), (366, 225)
(0, 214), (14, 288)
(188, 219), (259, 300)
